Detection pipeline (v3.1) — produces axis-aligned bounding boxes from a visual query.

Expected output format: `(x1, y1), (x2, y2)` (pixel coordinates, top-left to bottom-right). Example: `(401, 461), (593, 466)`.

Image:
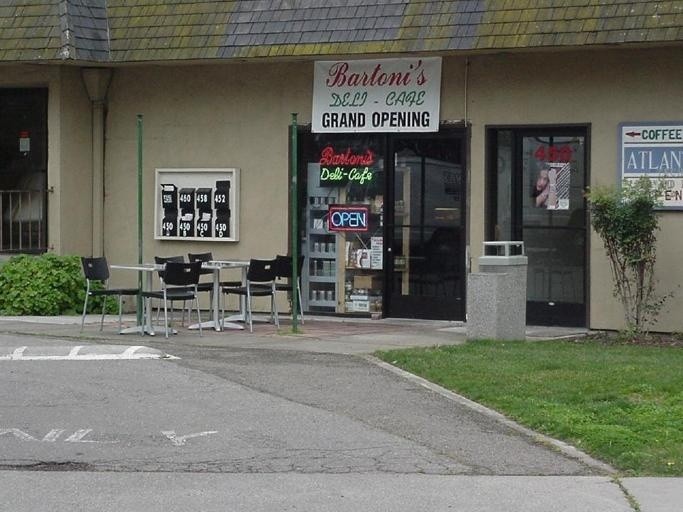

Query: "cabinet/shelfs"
(339, 166), (410, 314)
(301, 162), (339, 314)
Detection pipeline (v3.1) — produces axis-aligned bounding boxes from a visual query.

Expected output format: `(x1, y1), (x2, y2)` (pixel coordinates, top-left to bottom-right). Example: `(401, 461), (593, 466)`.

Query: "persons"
(530, 161), (560, 209)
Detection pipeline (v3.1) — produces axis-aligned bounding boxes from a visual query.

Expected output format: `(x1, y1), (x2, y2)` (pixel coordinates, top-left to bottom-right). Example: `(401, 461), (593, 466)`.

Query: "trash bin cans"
(466, 239), (527, 344)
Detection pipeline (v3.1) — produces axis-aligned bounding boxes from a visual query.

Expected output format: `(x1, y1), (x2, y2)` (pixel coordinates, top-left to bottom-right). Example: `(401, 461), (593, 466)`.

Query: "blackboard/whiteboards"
(329, 203), (371, 232)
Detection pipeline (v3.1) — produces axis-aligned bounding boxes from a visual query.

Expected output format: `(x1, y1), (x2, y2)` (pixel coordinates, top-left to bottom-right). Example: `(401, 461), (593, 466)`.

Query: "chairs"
(80, 252), (305, 339)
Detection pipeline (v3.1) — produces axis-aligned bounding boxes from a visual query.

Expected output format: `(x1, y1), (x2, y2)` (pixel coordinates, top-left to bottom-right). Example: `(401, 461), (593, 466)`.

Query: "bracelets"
(548, 183), (558, 193)
